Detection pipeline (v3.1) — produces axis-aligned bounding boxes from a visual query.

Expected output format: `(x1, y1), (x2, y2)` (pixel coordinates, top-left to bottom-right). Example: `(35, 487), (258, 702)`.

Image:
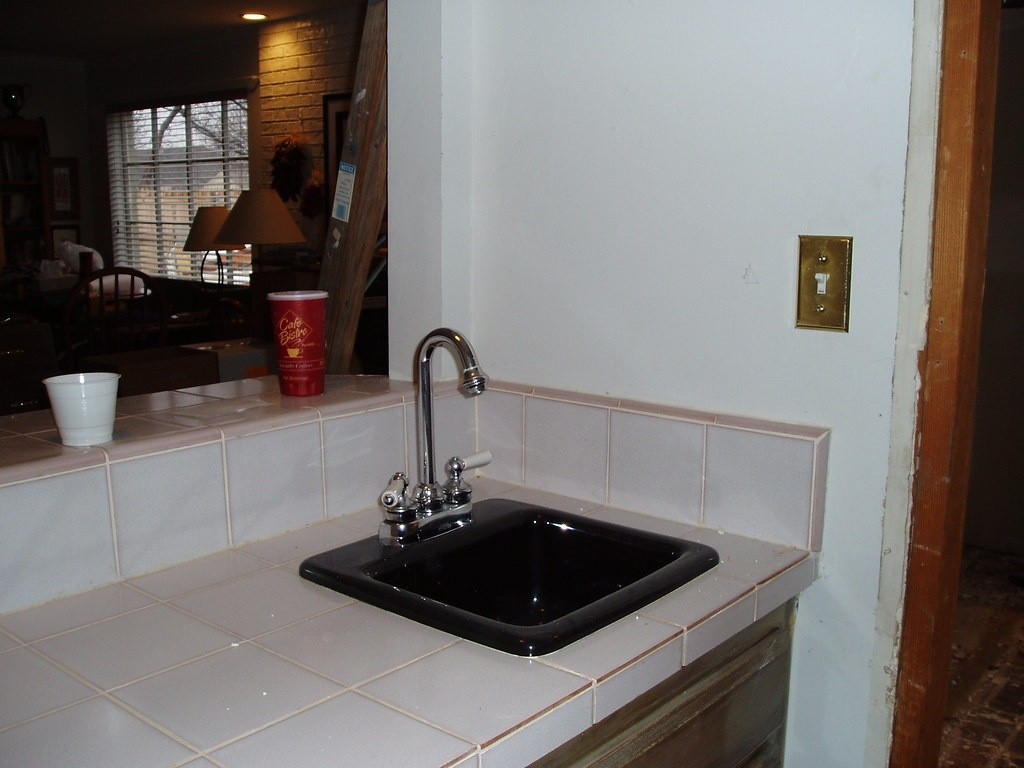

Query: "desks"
(0, 336), (368, 416)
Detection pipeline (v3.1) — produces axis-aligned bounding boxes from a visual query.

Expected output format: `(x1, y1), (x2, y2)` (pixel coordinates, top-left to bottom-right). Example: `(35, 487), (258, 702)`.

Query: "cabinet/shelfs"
(0, 116), (84, 275)
(524, 596), (794, 768)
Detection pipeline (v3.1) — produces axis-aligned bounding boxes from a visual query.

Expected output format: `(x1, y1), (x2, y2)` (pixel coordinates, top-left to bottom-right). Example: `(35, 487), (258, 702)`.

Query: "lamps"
(212, 188), (309, 345)
(183, 204), (245, 321)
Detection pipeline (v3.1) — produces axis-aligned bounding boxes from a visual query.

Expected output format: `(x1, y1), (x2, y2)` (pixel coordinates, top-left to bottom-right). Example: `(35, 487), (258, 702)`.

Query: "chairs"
(63, 267), (169, 357)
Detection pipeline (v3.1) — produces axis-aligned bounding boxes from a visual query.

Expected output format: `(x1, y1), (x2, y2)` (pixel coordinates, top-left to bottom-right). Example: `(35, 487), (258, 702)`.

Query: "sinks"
(348, 506), (722, 658)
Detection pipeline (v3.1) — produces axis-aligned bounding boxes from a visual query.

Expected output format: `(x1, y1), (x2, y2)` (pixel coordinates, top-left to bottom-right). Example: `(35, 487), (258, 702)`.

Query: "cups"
(42, 371), (122, 447)
(266, 290), (330, 399)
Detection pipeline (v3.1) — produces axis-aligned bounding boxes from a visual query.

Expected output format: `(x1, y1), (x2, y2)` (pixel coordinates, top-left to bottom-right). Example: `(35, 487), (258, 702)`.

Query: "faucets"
(409, 328), (490, 512)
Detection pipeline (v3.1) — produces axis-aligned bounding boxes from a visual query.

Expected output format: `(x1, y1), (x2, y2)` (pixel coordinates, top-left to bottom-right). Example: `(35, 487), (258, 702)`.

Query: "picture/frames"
(323, 93), (351, 234)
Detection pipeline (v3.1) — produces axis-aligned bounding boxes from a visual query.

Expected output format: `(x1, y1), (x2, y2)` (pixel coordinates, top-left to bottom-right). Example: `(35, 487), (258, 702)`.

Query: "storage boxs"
(41, 273), (76, 291)
